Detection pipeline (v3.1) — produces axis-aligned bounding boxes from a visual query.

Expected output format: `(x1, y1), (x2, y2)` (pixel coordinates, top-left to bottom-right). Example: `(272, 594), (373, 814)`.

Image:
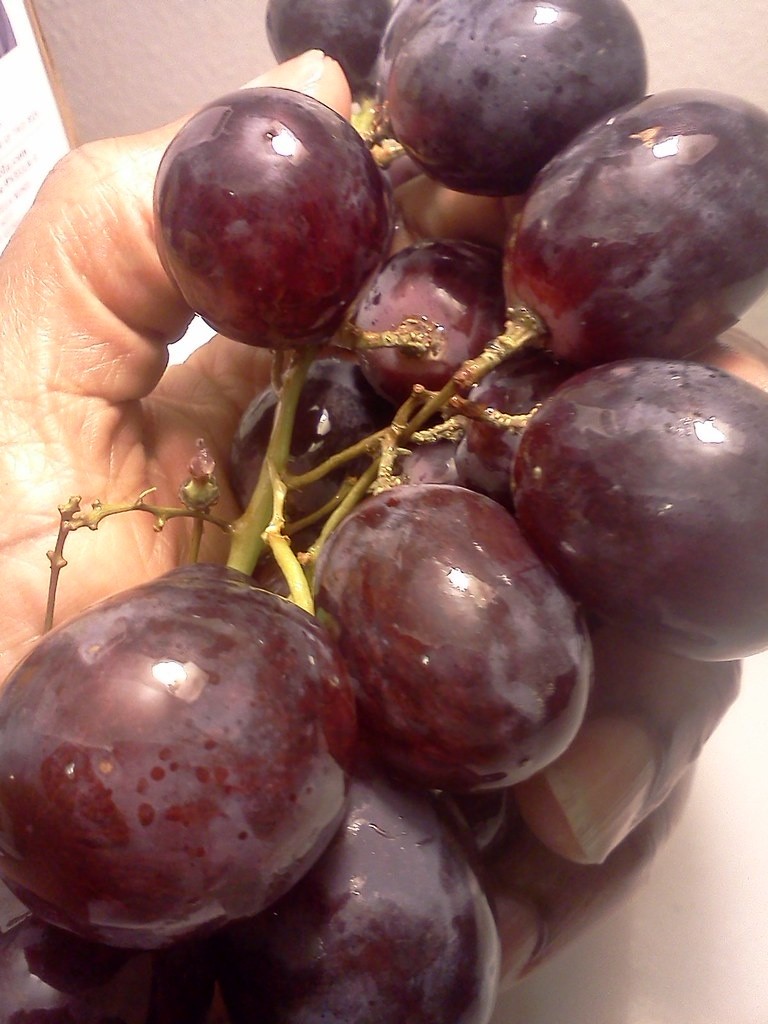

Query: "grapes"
(0, 0), (768, 1024)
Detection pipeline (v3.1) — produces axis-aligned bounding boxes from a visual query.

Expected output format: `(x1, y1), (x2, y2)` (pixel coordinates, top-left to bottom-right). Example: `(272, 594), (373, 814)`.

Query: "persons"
(0, 44), (767, 991)
